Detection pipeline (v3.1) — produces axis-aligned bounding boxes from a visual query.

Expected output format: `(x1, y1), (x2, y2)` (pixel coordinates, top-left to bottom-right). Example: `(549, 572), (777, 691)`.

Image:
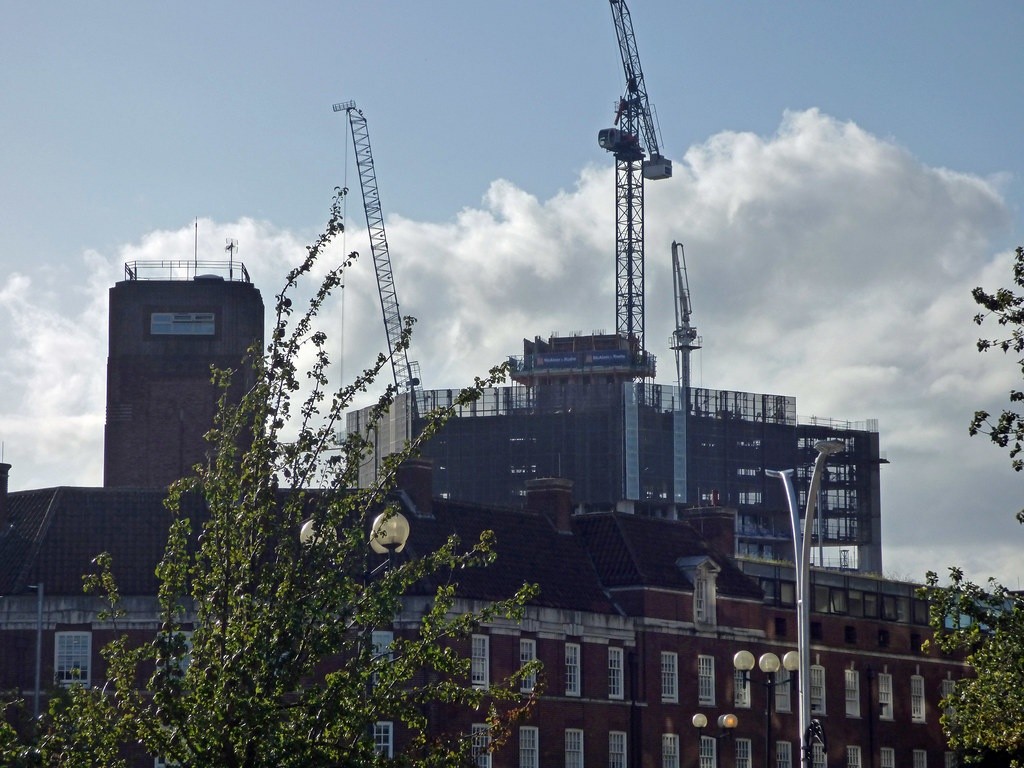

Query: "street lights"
(733, 648), (799, 768)
(692, 714), (739, 768)
(764, 439), (845, 768)
(301, 509), (412, 758)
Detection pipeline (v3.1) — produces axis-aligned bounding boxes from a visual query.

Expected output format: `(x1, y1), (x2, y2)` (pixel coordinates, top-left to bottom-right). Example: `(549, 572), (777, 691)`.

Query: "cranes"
(599, 0), (673, 354)
(332, 102), (427, 425)
(670, 241), (703, 413)
